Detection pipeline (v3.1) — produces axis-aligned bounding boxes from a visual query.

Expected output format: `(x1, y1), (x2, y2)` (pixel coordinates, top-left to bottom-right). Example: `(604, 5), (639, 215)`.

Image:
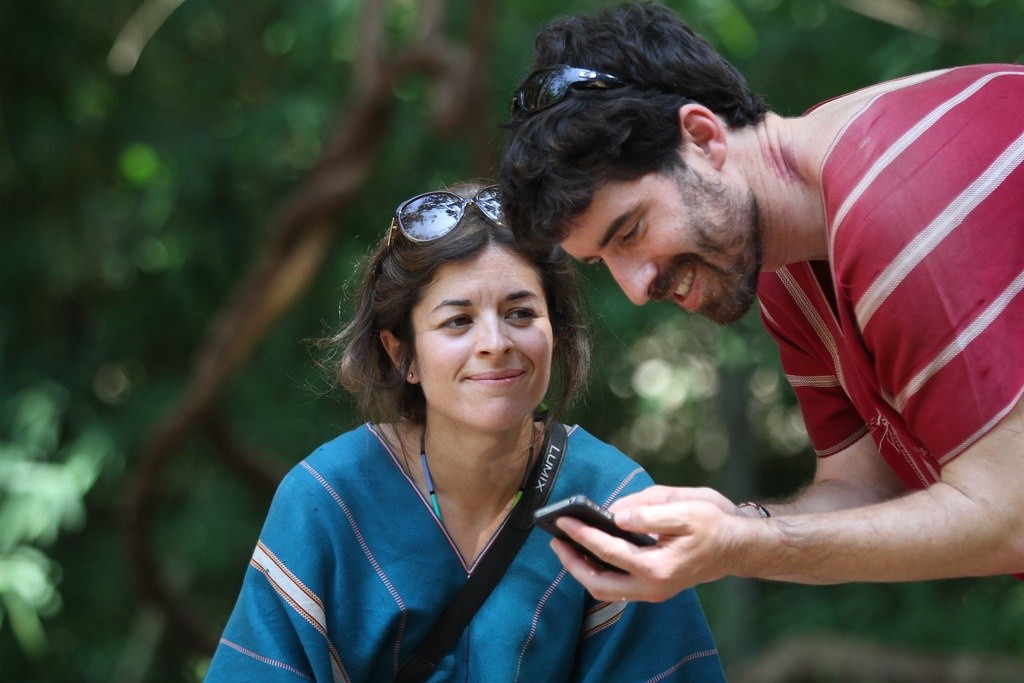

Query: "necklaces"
(421, 427), (534, 543)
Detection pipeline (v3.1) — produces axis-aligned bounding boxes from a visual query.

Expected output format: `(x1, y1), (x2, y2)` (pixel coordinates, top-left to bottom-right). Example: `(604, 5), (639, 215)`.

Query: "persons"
(203, 178), (726, 682)
(501, 0), (1024, 602)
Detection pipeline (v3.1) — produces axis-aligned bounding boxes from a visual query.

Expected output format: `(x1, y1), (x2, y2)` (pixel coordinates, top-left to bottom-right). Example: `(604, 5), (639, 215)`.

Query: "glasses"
(387, 183), (517, 255)
(509, 64), (633, 116)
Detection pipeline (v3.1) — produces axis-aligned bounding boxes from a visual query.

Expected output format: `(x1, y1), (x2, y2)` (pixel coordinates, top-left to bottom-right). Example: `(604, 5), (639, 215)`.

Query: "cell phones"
(534, 494), (658, 573)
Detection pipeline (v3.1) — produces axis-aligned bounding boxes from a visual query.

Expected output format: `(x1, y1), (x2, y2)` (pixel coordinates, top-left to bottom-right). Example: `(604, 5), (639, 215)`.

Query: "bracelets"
(738, 503), (770, 520)
